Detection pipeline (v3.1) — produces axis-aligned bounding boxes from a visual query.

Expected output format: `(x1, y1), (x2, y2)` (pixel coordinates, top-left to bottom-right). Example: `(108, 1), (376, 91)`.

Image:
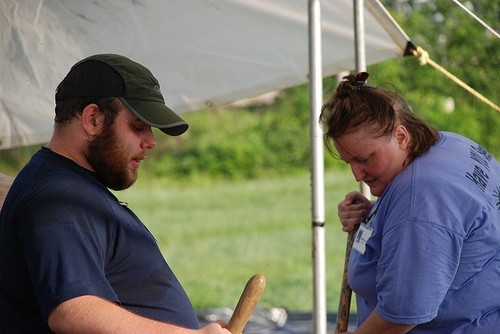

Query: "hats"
(54, 54), (189, 136)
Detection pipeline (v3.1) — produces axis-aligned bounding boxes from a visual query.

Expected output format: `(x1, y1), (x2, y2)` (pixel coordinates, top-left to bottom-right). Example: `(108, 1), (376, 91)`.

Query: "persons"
(0, 53), (237, 334)
(319, 69), (499, 334)
